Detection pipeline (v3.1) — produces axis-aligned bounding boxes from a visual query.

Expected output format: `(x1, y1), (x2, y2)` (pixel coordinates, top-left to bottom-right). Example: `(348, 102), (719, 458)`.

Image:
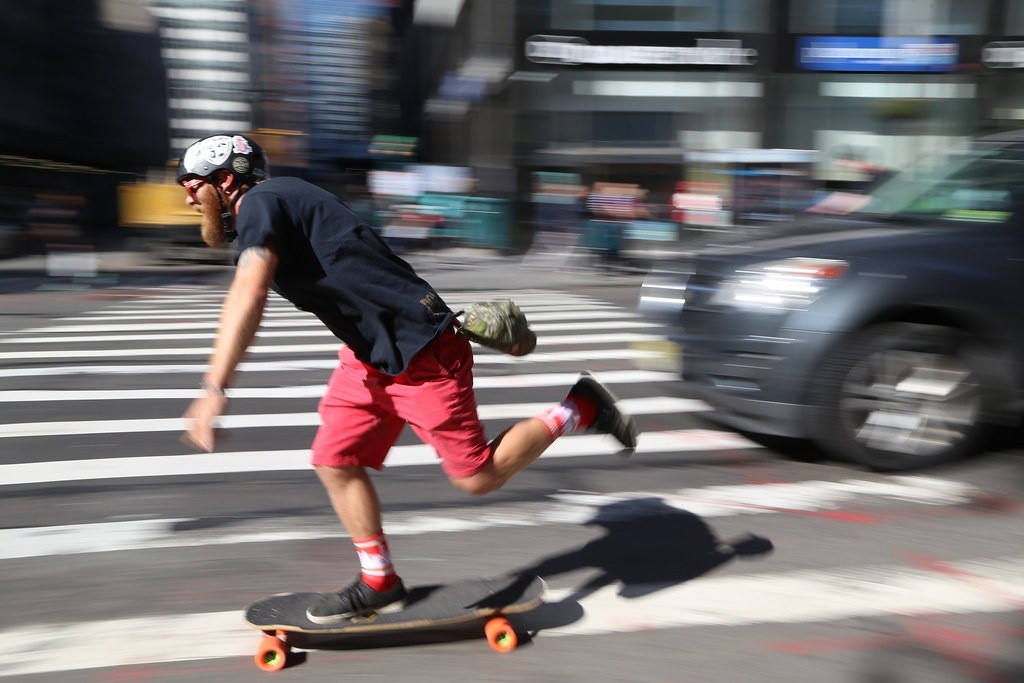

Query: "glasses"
(180, 178), (209, 203)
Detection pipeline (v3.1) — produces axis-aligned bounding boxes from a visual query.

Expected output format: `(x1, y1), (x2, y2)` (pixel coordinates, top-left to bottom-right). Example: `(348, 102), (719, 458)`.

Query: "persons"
(175, 131), (639, 623)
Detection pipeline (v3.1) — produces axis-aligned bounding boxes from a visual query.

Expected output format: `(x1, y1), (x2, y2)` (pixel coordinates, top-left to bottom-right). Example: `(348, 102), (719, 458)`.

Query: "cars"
(640, 130), (1024, 472)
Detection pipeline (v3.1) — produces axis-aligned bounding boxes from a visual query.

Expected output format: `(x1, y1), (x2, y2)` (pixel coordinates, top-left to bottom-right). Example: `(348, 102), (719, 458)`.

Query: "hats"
(453, 296), (538, 358)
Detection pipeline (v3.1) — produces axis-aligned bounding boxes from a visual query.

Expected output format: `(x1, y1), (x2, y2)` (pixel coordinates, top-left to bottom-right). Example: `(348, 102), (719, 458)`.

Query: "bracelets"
(196, 381), (227, 396)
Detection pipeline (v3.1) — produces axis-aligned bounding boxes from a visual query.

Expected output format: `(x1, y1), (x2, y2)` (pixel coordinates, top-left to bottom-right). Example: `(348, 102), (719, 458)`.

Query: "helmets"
(174, 133), (270, 187)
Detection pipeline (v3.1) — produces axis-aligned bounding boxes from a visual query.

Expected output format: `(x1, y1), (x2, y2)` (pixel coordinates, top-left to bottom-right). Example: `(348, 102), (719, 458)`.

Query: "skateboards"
(243, 574), (548, 672)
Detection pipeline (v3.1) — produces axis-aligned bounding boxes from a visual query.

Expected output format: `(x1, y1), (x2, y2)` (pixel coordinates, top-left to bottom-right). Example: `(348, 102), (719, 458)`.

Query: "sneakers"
(307, 571), (404, 626)
(563, 367), (638, 451)
